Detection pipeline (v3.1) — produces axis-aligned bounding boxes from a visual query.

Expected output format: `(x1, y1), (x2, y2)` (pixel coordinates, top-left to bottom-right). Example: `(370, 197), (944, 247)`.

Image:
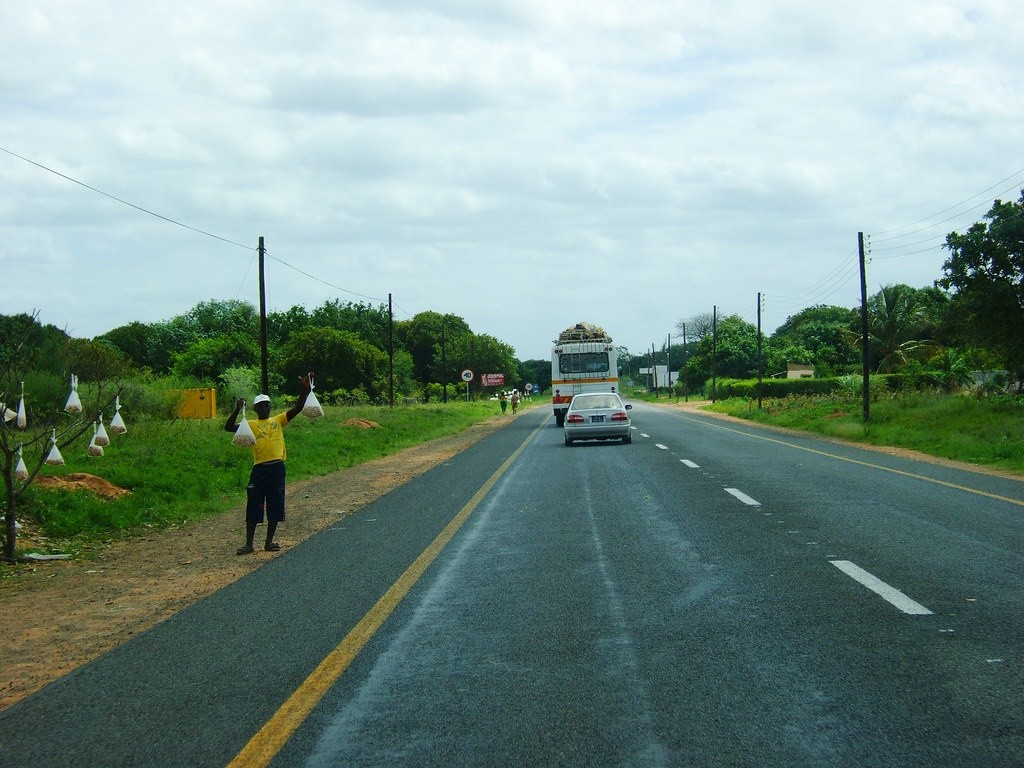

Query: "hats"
(254, 393), (270, 404)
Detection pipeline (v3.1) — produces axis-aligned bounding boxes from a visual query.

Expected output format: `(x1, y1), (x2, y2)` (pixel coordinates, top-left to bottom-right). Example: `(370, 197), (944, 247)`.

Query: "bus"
(552, 338), (620, 426)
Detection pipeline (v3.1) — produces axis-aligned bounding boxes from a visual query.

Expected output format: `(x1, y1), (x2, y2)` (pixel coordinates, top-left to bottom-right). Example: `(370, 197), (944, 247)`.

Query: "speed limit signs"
(526, 384), (533, 391)
(462, 370), (473, 382)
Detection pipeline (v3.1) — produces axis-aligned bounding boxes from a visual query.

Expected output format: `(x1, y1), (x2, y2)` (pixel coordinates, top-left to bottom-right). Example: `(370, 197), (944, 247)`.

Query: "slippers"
(236, 547), (254, 554)
(264, 543), (281, 551)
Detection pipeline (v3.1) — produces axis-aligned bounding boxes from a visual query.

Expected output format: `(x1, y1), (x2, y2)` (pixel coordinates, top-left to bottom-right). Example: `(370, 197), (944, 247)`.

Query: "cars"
(560, 393), (633, 445)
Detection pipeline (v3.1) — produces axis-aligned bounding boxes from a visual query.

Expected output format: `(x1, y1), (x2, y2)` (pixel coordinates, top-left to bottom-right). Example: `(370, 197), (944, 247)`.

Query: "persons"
(224, 373), (315, 555)
(499, 391), (518, 414)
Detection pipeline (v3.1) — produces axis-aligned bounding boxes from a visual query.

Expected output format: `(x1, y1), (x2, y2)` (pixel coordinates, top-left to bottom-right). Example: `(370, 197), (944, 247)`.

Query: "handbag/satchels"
(88, 420), (105, 458)
(64, 373), (82, 414)
(44, 429), (65, 466)
(17, 382), (26, 428)
(14, 442), (28, 482)
(230, 400), (258, 447)
(94, 412), (110, 447)
(110, 396), (127, 435)
(0, 402), (17, 422)
(302, 373), (324, 418)
